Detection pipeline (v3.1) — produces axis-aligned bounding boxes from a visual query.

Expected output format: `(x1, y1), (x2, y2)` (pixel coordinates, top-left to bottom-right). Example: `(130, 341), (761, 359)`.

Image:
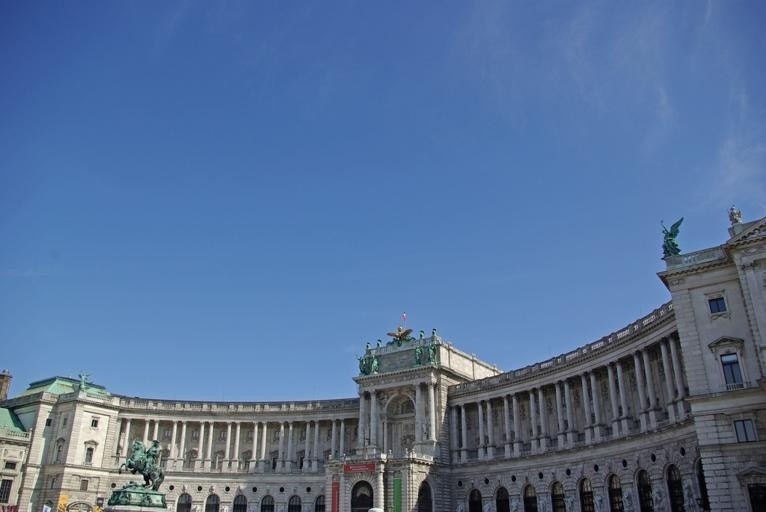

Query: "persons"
(728, 206), (743, 225)
(78, 372), (87, 389)
(356, 328), (437, 375)
(142, 439), (160, 475)
(660, 217), (684, 260)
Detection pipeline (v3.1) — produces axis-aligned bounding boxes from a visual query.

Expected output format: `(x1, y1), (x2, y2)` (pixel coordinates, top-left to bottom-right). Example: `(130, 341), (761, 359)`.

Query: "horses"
(118, 440), (164, 491)
